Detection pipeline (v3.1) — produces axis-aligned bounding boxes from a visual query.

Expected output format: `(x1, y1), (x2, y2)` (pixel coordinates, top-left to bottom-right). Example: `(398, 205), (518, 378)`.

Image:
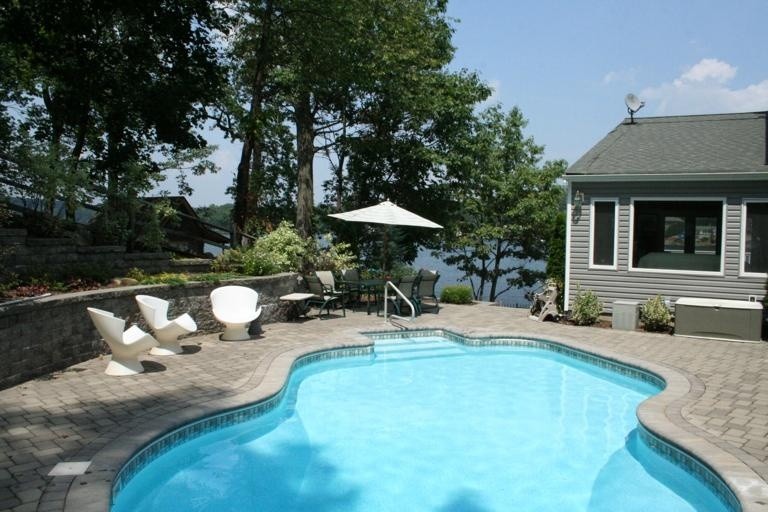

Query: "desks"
(280, 293), (315, 322)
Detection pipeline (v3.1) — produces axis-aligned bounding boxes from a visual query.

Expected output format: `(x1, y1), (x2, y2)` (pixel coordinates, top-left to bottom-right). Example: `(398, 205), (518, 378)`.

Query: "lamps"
(573, 190), (585, 210)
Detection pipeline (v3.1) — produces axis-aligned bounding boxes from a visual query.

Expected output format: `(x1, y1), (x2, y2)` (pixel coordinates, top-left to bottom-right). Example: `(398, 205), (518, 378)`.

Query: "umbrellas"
(327, 197), (444, 279)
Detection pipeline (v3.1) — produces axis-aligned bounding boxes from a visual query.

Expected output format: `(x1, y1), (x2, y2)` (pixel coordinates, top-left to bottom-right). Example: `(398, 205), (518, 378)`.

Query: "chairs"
(209, 285), (262, 341)
(86, 306), (160, 376)
(135, 294), (197, 356)
(304, 268), (441, 320)
